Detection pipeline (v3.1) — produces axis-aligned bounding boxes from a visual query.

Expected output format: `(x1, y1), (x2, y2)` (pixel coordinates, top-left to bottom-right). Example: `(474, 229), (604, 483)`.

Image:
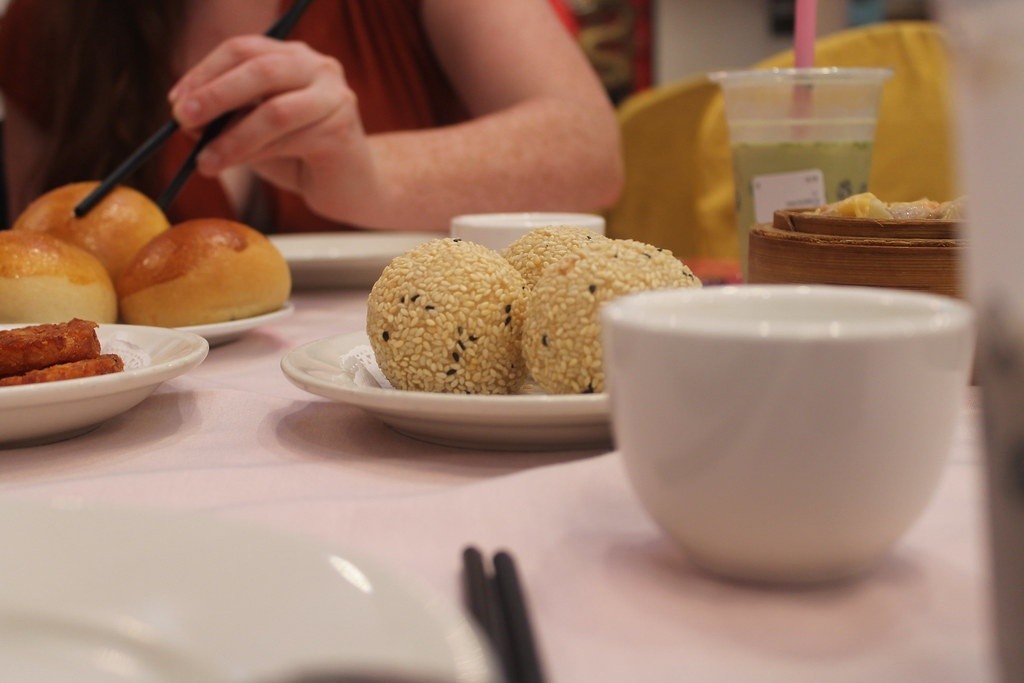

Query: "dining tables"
(1, 285), (1005, 683)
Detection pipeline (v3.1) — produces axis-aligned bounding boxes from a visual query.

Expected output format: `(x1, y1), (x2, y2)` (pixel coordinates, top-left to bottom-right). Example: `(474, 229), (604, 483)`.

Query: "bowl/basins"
(600, 284), (978, 594)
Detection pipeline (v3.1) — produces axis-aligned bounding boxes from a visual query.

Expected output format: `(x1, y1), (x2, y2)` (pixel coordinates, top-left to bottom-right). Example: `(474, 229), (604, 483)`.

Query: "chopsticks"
(71, 0), (312, 221)
(456, 543), (551, 683)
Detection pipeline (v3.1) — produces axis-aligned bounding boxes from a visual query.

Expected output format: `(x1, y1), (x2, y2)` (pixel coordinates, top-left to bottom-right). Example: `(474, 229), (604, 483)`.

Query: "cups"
(449, 212), (607, 259)
(706, 67), (893, 283)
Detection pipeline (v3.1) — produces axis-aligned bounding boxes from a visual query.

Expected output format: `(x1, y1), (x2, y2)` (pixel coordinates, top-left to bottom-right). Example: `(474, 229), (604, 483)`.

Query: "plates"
(277, 330), (613, 447)
(0, 323), (210, 452)
(266, 229), (452, 288)
(169, 296), (294, 348)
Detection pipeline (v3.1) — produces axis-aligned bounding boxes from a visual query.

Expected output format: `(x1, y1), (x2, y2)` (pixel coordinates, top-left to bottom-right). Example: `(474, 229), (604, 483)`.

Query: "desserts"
(522, 237), (703, 396)
(503, 226), (604, 288)
(366, 238), (530, 396)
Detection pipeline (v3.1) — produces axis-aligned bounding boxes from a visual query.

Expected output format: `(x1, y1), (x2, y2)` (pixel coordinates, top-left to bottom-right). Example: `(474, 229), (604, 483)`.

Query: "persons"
(0, 0), (626, 236)
